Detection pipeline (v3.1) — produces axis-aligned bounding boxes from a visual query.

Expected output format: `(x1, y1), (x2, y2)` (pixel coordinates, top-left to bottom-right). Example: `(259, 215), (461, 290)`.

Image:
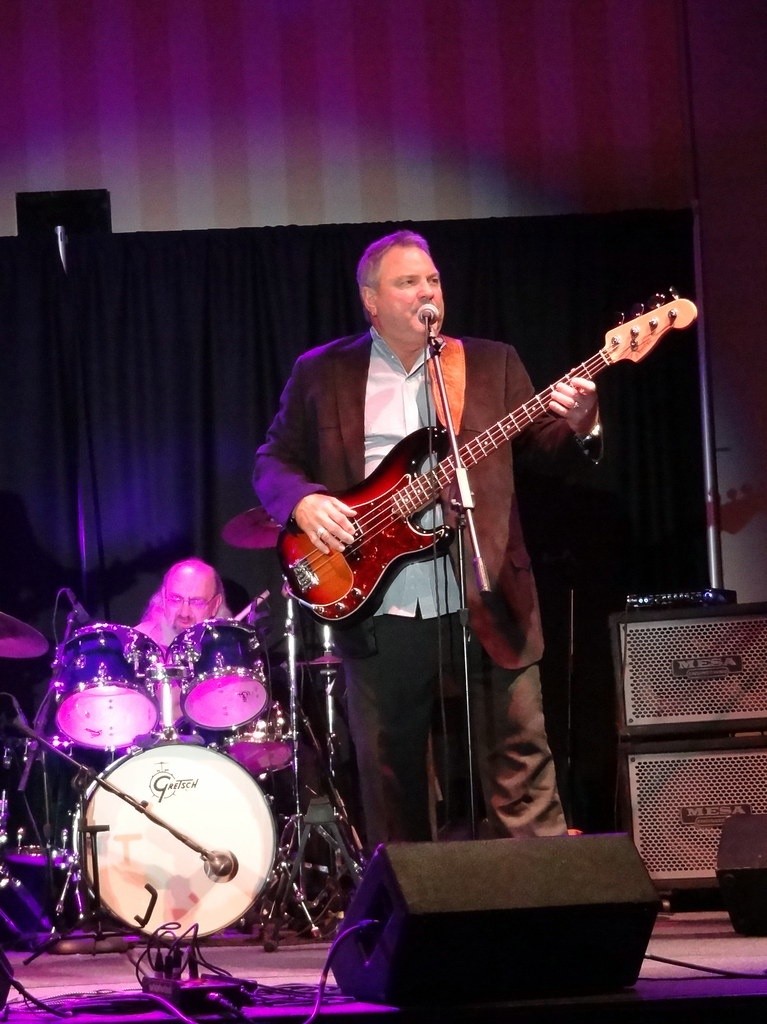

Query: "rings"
(573, 402), (579, 408)
(316, 528), (325, 537)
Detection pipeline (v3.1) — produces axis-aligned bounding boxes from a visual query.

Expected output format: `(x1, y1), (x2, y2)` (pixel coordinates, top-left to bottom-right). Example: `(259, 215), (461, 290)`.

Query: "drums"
(3, 734), (115, 868)
(166, 615), (270, 733)
(205, 703), (298, 777)
(55, 621), (165, 751)
(71, 739), (282, 942)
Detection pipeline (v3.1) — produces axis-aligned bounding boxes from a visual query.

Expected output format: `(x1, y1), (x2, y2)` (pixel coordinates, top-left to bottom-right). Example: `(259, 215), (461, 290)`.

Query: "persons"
(252, 232), (605, 853)
(132, 559), (232, 650)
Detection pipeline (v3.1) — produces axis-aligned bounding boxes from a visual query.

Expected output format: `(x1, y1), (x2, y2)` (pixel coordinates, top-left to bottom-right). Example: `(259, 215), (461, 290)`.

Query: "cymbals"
(0, 611), (49, 660)
(219, 504), (284, 551)
(285, 655), (342, 668)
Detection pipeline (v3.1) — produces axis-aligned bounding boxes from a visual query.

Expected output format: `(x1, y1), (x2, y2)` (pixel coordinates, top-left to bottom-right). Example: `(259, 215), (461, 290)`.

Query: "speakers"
(609, 602), (766, 939)
(326, 833), (659, 1003)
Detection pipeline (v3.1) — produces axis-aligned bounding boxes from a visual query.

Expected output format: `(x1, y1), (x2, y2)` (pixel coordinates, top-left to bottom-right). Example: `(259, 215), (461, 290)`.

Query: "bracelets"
(576, 425), (601, 446)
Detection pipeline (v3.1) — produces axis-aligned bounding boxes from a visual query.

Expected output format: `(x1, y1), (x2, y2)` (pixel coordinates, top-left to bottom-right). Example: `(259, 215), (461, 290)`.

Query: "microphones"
(11, 696), (28, 726)
(235, 589), (270, 622)
(66, 587), (90, 624)
(201, 850), (234, 877)
(417, 304), (439, 325)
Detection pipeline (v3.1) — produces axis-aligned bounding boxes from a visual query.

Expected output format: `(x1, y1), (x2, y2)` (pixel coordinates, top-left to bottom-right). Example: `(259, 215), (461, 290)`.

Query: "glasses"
(165, 594), (218, 610)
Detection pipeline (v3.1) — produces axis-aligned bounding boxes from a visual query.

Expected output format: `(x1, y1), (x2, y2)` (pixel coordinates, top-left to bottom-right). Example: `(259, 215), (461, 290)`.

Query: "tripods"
(240, 600), (364, 952)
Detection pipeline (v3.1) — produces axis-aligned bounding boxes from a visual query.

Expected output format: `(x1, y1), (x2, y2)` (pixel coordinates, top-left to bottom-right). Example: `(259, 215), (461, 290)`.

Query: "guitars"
(275, 285), (702, 630)
